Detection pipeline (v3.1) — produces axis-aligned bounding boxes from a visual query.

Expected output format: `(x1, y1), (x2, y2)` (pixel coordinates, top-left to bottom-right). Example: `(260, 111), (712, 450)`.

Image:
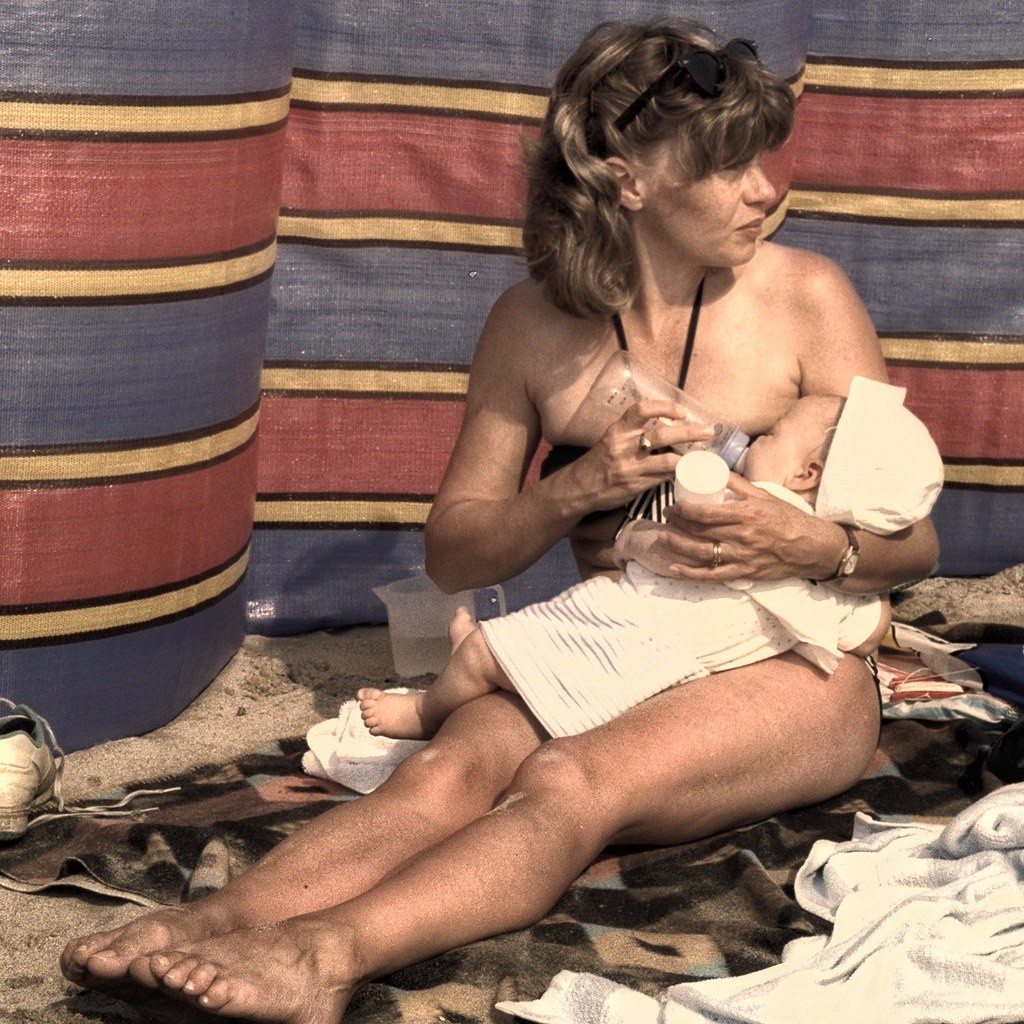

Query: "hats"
(814, 374), (945, 536)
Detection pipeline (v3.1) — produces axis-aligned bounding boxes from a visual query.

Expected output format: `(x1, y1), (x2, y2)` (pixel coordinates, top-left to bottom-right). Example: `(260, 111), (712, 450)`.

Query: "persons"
(61, 20), (940, 1024)
(356, 375), (944, 740)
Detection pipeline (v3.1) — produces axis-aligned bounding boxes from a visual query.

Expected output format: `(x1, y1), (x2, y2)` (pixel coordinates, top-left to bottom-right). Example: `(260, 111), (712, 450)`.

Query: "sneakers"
(0, 698), (65, 842)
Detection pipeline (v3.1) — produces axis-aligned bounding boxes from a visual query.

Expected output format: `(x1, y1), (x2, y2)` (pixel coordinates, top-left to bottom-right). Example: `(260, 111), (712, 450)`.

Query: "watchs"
(808, 525), (862, 587)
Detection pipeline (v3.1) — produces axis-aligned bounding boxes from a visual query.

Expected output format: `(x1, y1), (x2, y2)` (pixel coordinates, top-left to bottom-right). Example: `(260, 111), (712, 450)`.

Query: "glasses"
(593, 38), (760, 156)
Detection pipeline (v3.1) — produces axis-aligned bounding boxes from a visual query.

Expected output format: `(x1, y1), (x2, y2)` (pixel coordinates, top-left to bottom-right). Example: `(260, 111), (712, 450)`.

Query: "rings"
(711, 541), (722, 568)
(637, 432), (651, 457)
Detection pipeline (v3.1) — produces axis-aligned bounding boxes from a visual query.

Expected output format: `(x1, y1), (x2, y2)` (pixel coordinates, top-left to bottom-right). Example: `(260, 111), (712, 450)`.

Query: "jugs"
(370, 572), (506, 681)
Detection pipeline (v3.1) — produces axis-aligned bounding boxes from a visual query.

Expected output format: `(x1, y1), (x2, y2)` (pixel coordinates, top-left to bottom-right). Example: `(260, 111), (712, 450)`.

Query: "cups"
(673, 449), (729, 507)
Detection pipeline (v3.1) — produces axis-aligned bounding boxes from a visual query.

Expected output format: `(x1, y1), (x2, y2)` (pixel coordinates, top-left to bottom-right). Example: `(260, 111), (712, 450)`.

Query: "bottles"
(588, 349), (750, 471)
